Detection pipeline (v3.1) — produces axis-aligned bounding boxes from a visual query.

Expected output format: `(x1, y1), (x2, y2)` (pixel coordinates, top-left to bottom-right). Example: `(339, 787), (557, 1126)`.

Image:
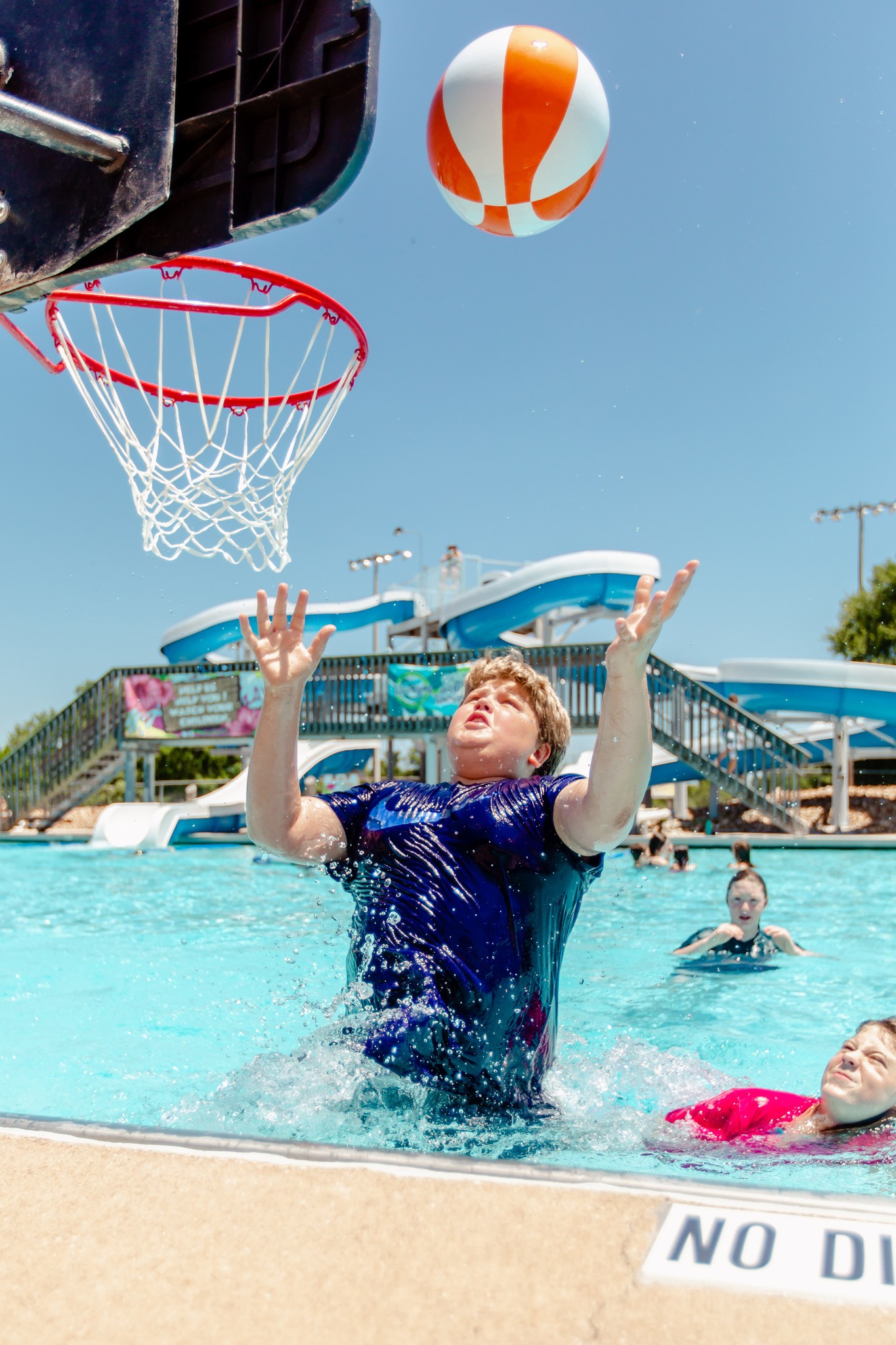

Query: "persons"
(728, 840), (756, 869)
(628, 832), (695, 873)
(671, 869), (815, 959)
(645, 1016), (896, 1158)
(242, 560), (701, 1118)
(440, 546), (462, 590)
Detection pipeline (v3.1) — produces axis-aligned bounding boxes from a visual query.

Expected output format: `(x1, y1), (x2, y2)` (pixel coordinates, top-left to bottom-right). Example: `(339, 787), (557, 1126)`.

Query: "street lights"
(813, 498), (896, 595)
(348, 550), (414, 655)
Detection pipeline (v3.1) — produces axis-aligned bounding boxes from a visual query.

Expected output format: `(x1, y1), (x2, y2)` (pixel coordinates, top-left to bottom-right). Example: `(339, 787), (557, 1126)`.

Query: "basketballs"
(426, 25), (611, 238)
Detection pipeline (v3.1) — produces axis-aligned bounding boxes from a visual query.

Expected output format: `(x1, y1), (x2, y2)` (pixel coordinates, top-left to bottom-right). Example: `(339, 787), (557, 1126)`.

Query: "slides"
(84, 589), (424, 852)
(437, 550), (896, 786)
